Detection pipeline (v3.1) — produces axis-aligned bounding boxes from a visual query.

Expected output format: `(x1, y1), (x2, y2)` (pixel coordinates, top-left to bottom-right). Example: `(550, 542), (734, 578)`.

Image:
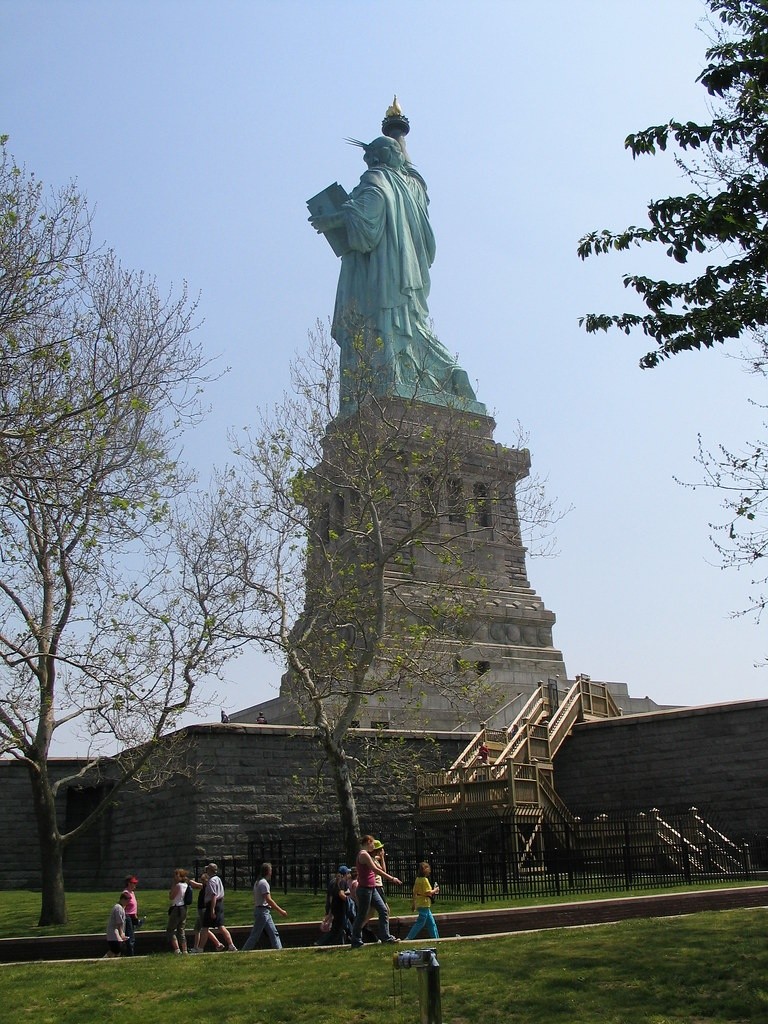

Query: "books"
(306, 181), (356, 257)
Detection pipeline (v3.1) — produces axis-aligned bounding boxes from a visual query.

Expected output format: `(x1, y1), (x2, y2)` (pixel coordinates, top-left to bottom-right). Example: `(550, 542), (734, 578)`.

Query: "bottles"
(136, 918), (145, 928)
(434, 882), (439, 894)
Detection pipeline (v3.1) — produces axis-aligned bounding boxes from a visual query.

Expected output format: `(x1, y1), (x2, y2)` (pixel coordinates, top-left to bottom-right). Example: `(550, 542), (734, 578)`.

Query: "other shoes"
(183, 950), (188, 954)
(175, 949), (181, 954)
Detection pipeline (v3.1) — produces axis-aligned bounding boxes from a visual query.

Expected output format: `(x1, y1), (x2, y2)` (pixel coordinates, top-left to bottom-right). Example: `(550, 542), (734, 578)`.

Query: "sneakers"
(382, 935), (401, 944)
(216, 943), (224, 952)
(226, 947), (237, 952)
(351, 936), (364, 947)
(190, 949), (204, 952)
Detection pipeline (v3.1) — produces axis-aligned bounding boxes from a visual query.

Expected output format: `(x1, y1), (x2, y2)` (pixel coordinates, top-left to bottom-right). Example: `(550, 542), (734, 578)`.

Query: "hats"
(339, 866), (351, 873)
(373, 840), (384, 849)
(130, 877), (138, 882)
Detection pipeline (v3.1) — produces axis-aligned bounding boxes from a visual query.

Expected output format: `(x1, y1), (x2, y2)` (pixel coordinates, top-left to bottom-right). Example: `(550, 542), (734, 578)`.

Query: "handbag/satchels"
(320, 911), (334, 933)
(417, 893), (436, 904)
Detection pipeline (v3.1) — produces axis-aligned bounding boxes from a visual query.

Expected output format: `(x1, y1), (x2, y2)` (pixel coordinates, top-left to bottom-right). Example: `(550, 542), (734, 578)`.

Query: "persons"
(221, 710), (231, 724)
(242, 863), (287, 950)
(405, 862), (439, 939)
(475, 741), (490, 781)
(256, 711), (267, 724)
(103, 875), (141, 958)
(314, 835), (402, 947)
(511, 725), (517, 738)
(308, 129), (476, 413)
(165, 863), (238, 953)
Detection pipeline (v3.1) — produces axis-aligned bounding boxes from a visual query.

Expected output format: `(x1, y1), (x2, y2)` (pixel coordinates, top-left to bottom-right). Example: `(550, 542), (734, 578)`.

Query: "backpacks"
(183, 886), (193, 905)
